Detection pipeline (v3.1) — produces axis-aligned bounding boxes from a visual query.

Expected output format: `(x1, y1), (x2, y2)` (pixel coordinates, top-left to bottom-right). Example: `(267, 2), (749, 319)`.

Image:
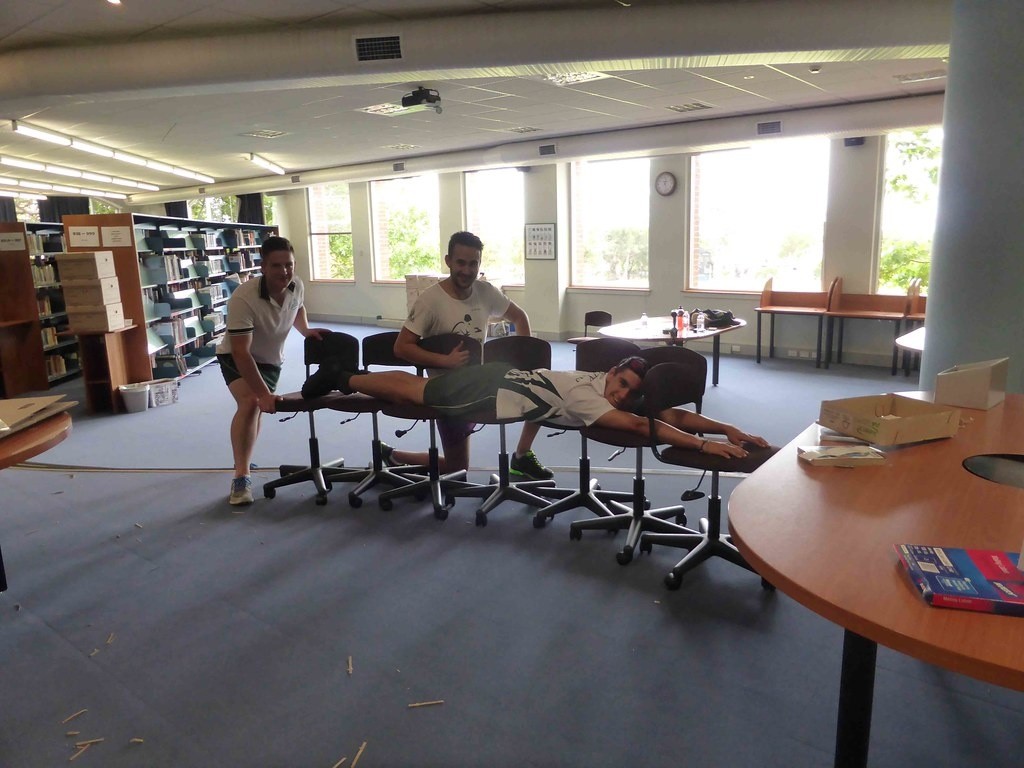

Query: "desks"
(598, 316), (746, 387)
(727, 391), (1024, 768)
(754, 277), (927, 378)
(0, 410), (72, 591)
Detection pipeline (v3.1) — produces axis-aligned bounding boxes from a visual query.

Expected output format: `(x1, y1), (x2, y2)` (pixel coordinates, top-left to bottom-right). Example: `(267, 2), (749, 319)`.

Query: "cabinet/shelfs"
(61, 212), (279, 415)
(0, 221), (83, 399)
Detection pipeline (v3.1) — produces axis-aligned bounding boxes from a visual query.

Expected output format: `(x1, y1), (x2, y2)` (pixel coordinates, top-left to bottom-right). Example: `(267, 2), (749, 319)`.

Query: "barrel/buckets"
(119, 383), (150, 413)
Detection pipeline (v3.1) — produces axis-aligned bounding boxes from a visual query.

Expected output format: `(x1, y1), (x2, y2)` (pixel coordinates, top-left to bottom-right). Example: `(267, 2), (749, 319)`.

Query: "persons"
(381, 232), (555, 481)
(216, 236), (332, 504)
(300, 356), (768, 459)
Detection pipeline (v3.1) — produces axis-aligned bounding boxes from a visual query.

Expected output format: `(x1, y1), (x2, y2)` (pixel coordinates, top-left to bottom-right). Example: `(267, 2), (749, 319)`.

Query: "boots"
(301, 353), (370, 400)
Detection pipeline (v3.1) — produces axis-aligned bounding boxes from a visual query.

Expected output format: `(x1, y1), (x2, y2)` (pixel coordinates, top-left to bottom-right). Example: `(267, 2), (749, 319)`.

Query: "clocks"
(655, 171), (676, 197)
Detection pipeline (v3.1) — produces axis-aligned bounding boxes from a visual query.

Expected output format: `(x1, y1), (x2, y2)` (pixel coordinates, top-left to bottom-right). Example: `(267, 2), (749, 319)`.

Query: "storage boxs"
(141, 378), (179, 409)
(55, 251), (125, 332)
(935, 357), (1010, 411)
(819, 393), (960, 446)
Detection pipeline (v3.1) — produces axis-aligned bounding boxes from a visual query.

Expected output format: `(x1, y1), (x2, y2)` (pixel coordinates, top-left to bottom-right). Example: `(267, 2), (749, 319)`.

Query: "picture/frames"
(524, 223), (556, 260)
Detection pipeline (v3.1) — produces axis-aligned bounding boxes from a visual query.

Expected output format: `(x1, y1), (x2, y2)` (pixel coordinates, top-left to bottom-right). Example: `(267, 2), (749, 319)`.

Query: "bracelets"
(700, 440), (707, 450)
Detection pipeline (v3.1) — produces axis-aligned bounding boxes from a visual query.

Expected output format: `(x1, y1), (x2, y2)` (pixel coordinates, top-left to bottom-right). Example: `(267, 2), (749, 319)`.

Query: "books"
(31, 264), (54, 285)
(41, 327), (58, 349)
(36, 295), (51, 316)
(798, 446), (884, 467)
(27, 234), (43, 253)
(45, 354), (66, 381)
(142, 229), (256, 375)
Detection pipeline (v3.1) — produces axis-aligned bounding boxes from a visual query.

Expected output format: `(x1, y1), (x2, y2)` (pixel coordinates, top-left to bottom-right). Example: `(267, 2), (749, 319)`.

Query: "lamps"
(0, 119), (216, 200)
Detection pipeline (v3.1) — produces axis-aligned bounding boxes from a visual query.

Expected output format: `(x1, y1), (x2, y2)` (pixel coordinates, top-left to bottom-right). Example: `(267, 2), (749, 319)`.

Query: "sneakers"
(509, 449), (555, 481)
(381, 442), (405, 467)
(229, 476), (255, 504)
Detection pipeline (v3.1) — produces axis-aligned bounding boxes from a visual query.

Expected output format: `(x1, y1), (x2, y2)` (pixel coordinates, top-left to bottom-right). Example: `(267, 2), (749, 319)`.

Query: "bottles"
(696, 312), (705, 331)
(676, 305), (690, 331)
(641, 313), (648, 326)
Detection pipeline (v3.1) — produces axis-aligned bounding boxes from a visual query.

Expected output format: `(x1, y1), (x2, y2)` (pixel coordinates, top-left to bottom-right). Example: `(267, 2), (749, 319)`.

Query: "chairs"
(263, 332), (777, 594)
(566, 310), (612, 352)
